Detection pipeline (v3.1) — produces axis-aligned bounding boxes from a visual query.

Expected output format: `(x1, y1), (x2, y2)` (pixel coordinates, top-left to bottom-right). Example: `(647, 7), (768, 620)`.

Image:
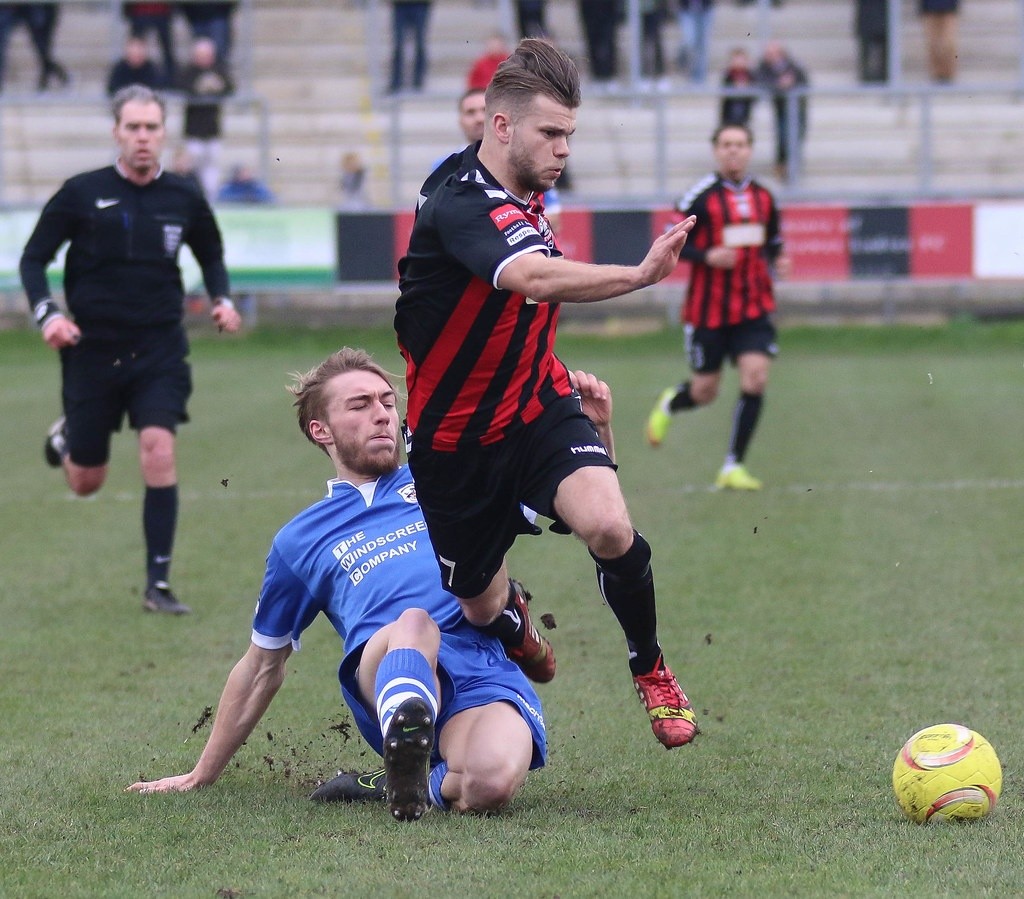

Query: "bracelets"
(215, 298), (232, 305)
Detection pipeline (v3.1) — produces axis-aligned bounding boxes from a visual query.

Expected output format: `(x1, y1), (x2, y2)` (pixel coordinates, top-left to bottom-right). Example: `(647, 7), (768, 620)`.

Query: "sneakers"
(46, 416), (74, 468)
(139, 588), (191, 620)
(645, 385), (680, 449)
(309, 764), (383, 801)
(384, 697), (434, 822)
(631, 660), (703, 751)
(712, 468), (767, 490)
(509, 578), (555, 683)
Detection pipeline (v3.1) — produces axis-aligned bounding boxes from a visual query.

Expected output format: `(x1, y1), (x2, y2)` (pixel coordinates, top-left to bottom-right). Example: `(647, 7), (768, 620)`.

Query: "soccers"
(892, 724), (1003, 823)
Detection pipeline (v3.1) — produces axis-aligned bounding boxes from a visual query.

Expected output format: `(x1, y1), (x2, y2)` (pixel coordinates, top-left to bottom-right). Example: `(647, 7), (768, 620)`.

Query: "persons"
(23, 0), (70, 89)
(218, 161), (276, 205)
(511, 0), (547, 39)
(183, 36), (236, 190)
(721, 48), (755, 128)
(387, 0), (430, 92)
(20, 83), (241, 616)
(181, 0), (239, 59)
(578, 0), (624, 80)
(468, 33), (512, 89)
(431, 88), (560, 233)
(918, 0), (959, 80)
(852, 0), (887, 84)
(339, 150), (364, 192)
(393, 39), (699, 750)
(109, 38), (162, 92)
(642, 121), (790, 490)
(121, 347), (548, 821)
(758, 42), (809, 166)
(641, 0), (714, 81)
(120, 0), (177, 69)
